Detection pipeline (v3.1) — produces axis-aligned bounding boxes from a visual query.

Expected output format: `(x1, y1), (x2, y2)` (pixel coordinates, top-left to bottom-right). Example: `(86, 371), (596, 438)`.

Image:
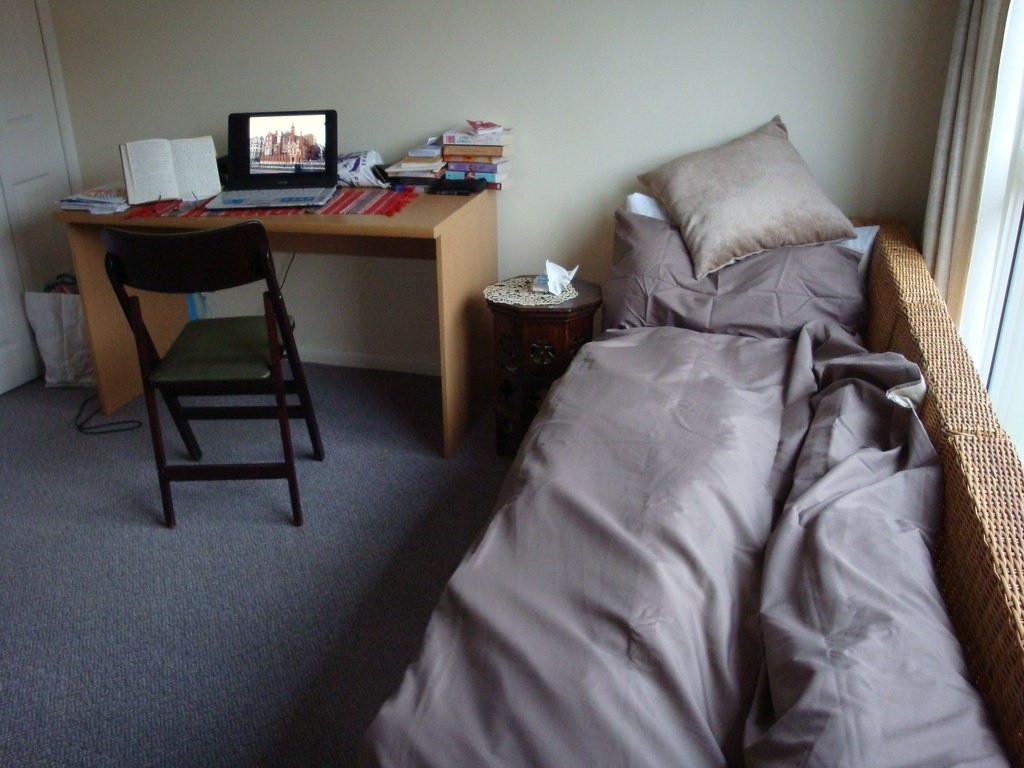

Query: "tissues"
(529, 259), (580, 295)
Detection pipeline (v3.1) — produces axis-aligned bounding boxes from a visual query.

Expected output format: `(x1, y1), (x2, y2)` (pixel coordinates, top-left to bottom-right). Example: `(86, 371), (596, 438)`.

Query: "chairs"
(104, 221), (325, 530)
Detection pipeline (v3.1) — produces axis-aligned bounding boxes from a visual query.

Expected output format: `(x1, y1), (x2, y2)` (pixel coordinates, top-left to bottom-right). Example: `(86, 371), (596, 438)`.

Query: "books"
(118, 135), (223, 206)
(384, 120), (513, 192)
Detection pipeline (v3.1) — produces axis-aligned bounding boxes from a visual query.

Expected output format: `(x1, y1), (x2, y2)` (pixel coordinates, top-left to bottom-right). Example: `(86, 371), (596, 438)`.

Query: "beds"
(344, 215), (1024, 768)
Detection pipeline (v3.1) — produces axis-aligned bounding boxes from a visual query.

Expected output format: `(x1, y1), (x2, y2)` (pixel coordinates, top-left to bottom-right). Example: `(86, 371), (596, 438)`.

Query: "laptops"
(205, 109), (338, 209)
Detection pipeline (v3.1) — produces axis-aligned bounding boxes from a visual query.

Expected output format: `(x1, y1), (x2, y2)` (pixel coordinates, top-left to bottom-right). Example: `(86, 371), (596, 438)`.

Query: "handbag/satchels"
(21, 290), (99, 392)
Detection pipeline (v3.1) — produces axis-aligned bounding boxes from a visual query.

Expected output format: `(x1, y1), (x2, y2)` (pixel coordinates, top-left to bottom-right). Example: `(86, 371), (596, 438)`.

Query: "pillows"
(625, 192), (880, 280)
(638, 113), (858, 281)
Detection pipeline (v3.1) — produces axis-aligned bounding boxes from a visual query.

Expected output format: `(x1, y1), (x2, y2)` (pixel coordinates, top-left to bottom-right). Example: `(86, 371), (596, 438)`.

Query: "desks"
(51, 184), (502, 463)
(480, 273), (602, 456)
